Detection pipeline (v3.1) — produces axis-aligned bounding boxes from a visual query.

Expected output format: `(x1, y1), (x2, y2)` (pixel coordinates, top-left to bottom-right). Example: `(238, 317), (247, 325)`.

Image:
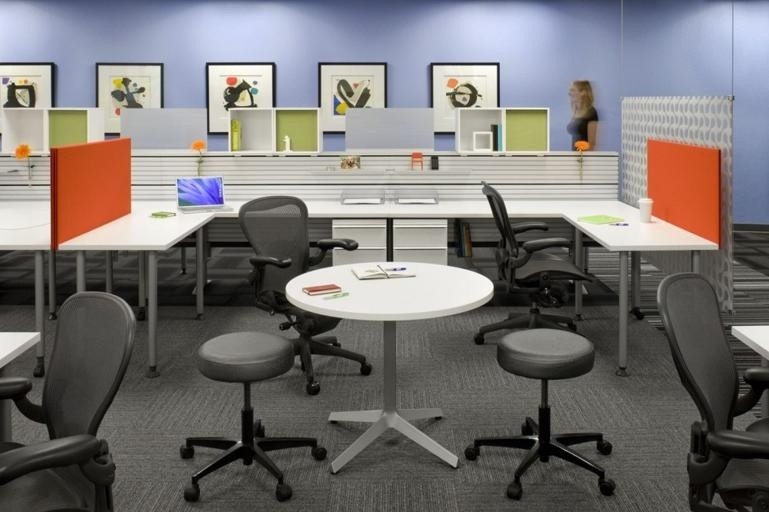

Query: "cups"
(638, 198), (654, 223)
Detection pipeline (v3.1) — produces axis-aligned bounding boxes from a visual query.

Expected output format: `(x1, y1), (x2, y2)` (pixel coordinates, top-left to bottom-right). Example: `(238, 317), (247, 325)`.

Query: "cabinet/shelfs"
(0, 107), (105, 156)
(454, 107), (550, 155)
(227, 107), (323, 153)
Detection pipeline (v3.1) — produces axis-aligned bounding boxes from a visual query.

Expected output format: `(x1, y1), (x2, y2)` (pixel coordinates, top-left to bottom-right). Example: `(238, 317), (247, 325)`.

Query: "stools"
(459, 325), (622, 502)
(174, 329), (327, 502)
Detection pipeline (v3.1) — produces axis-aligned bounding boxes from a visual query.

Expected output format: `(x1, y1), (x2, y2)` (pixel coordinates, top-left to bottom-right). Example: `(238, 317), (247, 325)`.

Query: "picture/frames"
(0, 61), (55, 110)
(205, 60), (277, 136)
(94, 61), (165, 137)
(317, 61), (387, 135)
(430, 62), (500, 136)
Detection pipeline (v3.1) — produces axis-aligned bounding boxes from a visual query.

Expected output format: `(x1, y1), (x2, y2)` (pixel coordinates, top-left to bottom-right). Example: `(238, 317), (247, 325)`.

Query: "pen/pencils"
(610, 223), (629, 226)
(386, 267), (406, 271)
(323, 293), (349, 299)
(377, 264), (385, 273)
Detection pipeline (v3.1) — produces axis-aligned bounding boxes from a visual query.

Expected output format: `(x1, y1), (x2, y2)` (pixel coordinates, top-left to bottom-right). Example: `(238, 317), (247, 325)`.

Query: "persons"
(565, 79), (599, 151)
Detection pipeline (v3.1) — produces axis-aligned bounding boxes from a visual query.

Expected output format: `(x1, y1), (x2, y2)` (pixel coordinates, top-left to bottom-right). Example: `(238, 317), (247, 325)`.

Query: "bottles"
(411, 152), (423, 171)
(231, 118), (241, 152)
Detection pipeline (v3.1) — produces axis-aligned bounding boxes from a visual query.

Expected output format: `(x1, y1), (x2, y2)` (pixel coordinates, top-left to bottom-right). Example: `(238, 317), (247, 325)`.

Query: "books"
(351, 264), (416, 280)
(302, 284), (341, 295)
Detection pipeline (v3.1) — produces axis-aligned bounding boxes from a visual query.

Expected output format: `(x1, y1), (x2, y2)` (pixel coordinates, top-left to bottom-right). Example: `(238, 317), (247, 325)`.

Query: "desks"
(391, 199), (713, 379)
(284, 258), (497, 475)
(0, 200), (55, 374)
(51, 198), (390, 380)
(728, 321), (769, 418)
(1, 329), (42, 445)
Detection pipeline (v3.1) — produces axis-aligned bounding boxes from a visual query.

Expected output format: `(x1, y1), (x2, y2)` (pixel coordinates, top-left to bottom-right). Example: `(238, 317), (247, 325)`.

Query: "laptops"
(176, 176), (234, 213)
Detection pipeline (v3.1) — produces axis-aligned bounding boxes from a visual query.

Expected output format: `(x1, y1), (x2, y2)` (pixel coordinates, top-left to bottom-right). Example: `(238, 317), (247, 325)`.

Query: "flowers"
(190, 139), (206, 176)
(15, 145), (36, 178)
(574, 139), (590, 168)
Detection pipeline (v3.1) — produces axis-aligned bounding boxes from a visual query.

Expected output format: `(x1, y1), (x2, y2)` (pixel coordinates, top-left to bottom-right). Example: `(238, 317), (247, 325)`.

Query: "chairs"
(469, 180), (593, 358)
(234, 195), (371, 399)
(655, 270), (768, 511)
(0, 290), (144, 511)
